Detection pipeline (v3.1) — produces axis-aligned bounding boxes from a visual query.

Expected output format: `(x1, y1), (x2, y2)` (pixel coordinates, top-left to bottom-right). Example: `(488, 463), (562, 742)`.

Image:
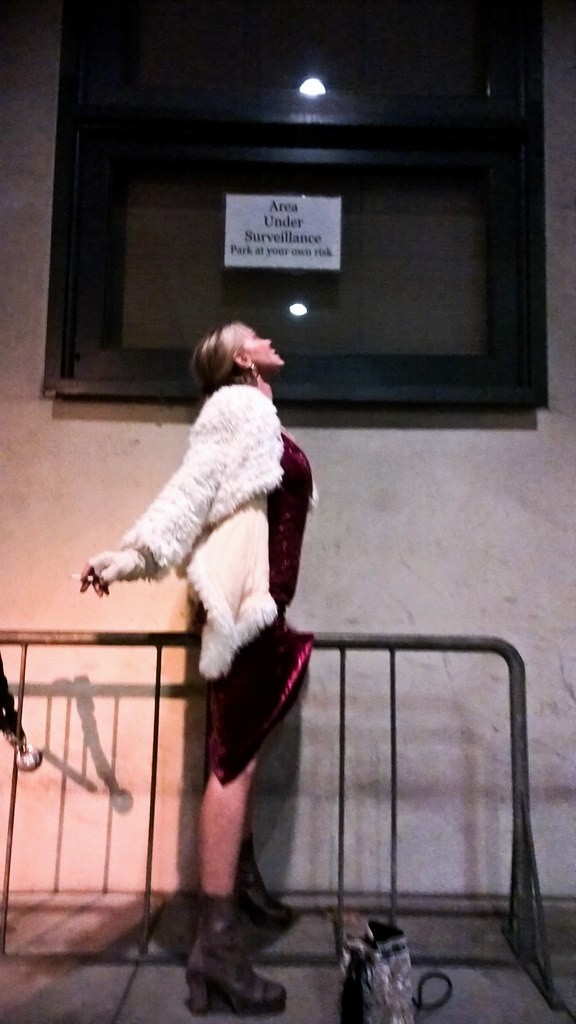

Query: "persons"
(80, 323), (318, 1013)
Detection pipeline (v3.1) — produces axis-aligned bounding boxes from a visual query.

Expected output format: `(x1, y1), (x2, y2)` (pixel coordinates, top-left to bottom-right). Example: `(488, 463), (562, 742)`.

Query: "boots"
(186, 894), (285, 1010)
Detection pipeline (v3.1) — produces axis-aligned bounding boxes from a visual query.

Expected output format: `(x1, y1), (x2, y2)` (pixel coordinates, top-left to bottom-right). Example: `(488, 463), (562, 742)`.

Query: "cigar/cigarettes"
(70, 574), (94, 581)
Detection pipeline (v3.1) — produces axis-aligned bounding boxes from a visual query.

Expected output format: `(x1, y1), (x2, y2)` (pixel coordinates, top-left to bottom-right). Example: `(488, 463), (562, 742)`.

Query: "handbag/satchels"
(337, 919), (450, 1024)
